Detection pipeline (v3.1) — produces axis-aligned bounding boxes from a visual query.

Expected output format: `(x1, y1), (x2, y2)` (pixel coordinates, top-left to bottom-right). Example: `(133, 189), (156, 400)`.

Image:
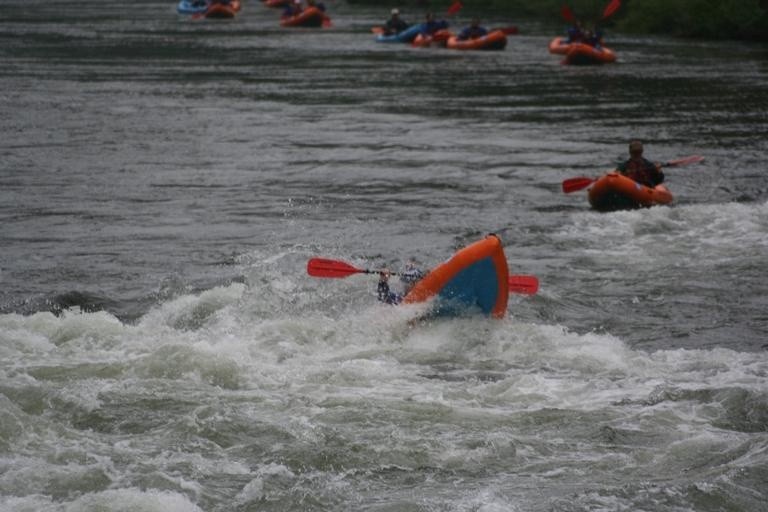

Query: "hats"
(629, 141), (642, 152)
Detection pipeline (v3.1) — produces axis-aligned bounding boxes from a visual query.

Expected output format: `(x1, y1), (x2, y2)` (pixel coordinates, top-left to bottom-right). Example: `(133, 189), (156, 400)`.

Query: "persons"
(615, 140), (664, 189)
(377, 263), (426, 305)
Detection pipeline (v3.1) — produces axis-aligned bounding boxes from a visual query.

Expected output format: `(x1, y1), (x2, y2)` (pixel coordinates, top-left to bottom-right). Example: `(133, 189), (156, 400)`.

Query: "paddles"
(563, 156), (705, 193)
(489, 26), (518, 34)
(441, 2), (462, 20)
(595, 1), (620, 24)
(561, 6), (575, 21)
(308, 259), (538, 294)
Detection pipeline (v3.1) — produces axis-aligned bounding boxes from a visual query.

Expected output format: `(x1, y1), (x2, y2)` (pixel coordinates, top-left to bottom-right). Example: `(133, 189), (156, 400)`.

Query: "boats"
(413, 29), (449, 47)
(561, 43), (614, 68)
(373, 19), (448, 44)
(398, 235), (510, 320)
(547, 35), (571, 53)
(177, 0), (329, 27)
(447, 29), (508, 50)
(586, 174), (674, 213)
